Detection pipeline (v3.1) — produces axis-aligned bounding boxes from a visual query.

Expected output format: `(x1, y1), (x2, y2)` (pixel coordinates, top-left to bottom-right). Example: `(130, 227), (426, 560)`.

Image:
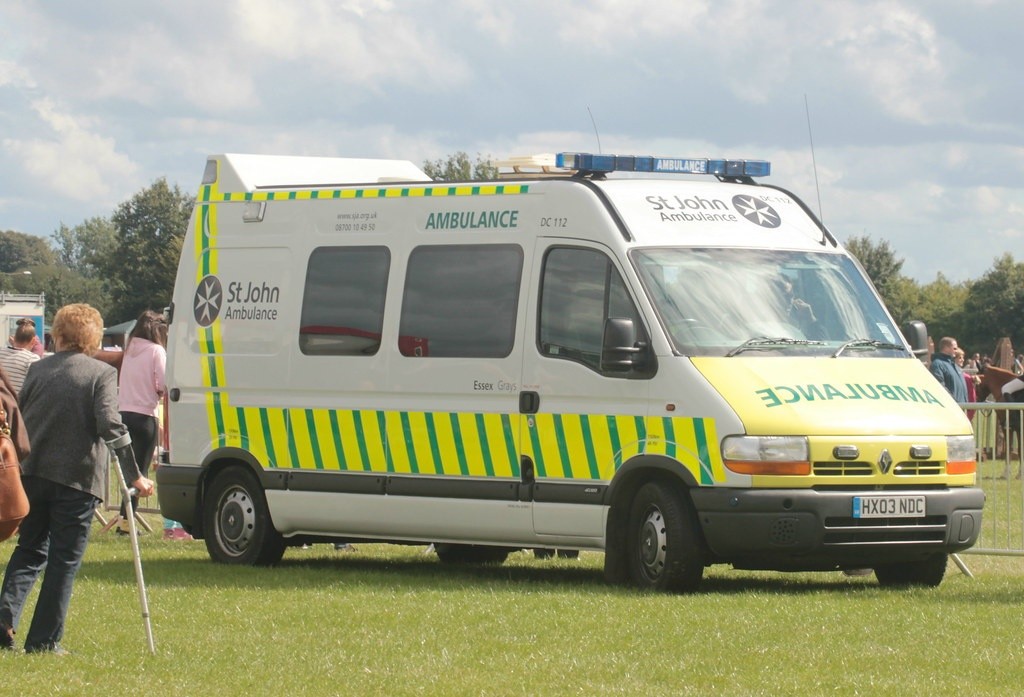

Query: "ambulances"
(156, 146), (987, 597)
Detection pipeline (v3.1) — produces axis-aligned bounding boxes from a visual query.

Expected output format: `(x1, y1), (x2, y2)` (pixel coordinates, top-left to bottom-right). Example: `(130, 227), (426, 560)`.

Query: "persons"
(759, 274), (831, 341)
(0, 303), (199, 654)
(929, 337), (1024, 422)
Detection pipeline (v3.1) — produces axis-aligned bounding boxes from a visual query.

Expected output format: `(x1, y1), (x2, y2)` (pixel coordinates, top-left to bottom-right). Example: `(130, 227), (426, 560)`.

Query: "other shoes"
(0, 622), (15, 650)
(116, 523), (143, 536)
(163, 528), (174, 539)
(334, 544), (356, 553)
(26, 642), (71, 656)
(174, 528), (192, 540)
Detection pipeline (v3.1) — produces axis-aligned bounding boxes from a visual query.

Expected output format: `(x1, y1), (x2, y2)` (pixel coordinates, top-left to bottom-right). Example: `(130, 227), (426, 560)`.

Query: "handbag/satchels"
(0, 399), (30, 543)
